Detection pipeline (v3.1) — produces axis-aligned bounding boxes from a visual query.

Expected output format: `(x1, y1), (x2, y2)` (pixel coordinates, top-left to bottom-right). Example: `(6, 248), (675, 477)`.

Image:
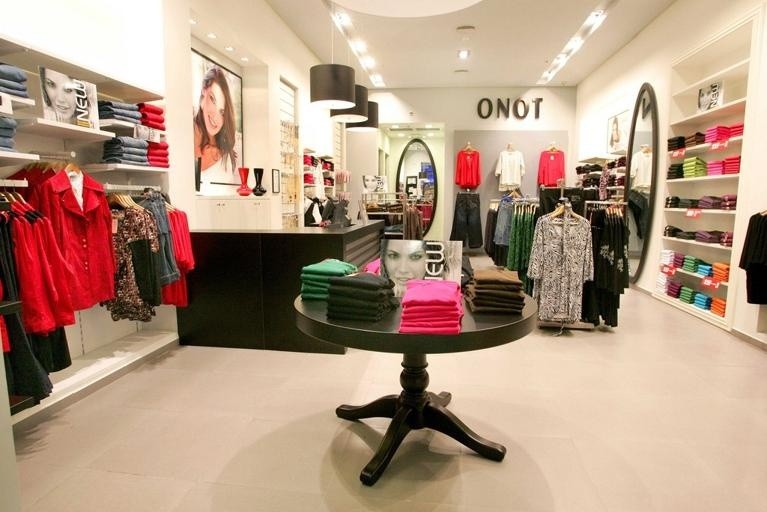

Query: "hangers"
(487, 178), (630, 224)
(463, 143), (560, 154)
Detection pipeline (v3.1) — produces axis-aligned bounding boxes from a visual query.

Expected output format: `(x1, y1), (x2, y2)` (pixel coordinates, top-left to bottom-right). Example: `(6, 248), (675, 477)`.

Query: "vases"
(235, 167), (266, 197)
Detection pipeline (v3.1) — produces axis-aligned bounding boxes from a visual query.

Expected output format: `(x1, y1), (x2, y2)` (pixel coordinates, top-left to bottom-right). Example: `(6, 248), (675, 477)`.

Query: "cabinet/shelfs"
(652, 12), (762, 333)
(578, 149), (625, 190)
(303, 147), (335, 189)
(0, 38), (178, 431)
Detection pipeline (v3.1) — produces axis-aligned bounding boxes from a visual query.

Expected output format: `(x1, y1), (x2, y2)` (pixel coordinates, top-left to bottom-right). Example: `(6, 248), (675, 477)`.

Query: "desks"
(295, 271), (539, 487)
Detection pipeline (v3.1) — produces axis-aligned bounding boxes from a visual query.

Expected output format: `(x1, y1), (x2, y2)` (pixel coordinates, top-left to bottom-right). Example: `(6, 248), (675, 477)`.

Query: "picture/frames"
(191, 46), (244, 186)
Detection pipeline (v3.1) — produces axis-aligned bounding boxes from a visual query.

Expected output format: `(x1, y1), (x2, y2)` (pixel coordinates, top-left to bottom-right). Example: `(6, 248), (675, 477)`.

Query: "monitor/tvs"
(321, 199), (352, 226)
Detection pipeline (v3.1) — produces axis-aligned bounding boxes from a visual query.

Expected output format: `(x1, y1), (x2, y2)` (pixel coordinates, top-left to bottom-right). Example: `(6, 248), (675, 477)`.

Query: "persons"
(381, 239), (448, 298)
(193, 65), (237, 187)
(41, 66), (90, 125)
(362, 175), (382, 192)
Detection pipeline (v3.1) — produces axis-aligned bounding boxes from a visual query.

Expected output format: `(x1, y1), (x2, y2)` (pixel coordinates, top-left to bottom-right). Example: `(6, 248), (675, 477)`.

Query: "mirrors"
(622, 82), (660, 284)
(395, 139), (437, 238)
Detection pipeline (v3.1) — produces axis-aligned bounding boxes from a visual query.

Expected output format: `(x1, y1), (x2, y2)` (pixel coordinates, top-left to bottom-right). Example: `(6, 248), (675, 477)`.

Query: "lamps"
(309, 0), (378, 132)
